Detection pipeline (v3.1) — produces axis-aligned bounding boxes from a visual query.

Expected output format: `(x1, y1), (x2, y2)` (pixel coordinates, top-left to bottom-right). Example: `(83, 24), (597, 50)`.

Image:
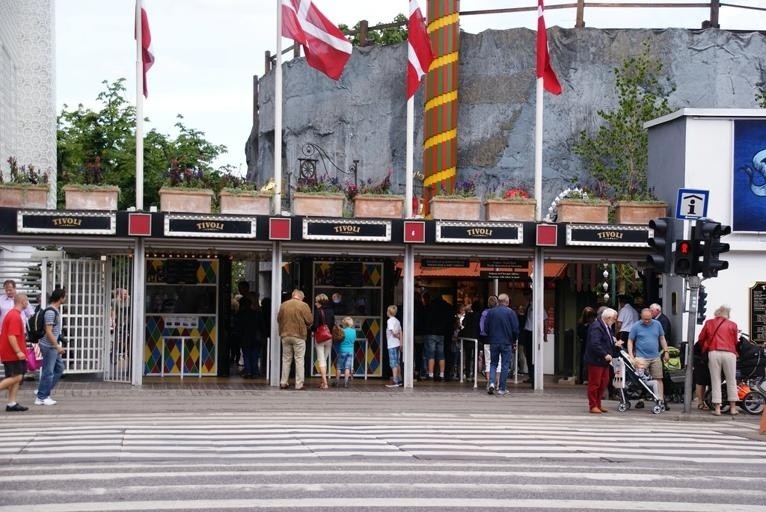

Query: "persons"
(692, 342), (708, 410)
(698, 307), (740, 416)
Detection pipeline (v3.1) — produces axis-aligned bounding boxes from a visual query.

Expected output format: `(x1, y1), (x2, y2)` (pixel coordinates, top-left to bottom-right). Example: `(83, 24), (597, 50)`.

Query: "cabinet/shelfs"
(312, 260), (382, 318)
(145, 256), (218, 316)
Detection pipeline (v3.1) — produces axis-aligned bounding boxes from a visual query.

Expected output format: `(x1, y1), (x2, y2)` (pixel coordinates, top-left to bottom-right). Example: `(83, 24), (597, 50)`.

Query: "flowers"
(291, 175), (358, 200)
(485, 186), (535, 201)
(428, 179), (482, 202)
(543, 178), (611, 223)
(219, 162), (275, 195)
(340, 164), (405, 199)
(618, 178), (661, 200)
(61, 155), (124, 199)
(1, 158), (50, 187)
(162, 157), (215, 192)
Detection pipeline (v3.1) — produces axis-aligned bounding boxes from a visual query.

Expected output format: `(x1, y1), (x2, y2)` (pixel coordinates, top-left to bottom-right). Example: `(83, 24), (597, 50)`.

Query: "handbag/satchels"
(332, 324), (344, 344)
(32, 342), (43, 360)
(315, 324), (333, 343)
(702, 352), (708, 362)
(26, 348), (42, 371)
(463, 317), (475, 338)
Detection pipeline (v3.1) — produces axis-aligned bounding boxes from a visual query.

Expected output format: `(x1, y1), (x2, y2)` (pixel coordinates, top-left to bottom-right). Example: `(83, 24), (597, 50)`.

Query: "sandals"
(698, 403), (709, 410)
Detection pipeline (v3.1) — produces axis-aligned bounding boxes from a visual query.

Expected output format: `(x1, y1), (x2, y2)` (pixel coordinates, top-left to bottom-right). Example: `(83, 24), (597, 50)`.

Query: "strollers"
(701, 335), (766, 417)
(609, 339), (666, 415)
(657, 343), (693, 403)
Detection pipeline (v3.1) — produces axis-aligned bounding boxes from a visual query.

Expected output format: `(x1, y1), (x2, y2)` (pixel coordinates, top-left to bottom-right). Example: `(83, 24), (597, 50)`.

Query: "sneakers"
(33, 389), (57, 406)
(486, 383), (509, 395)
(318, 380), (348, 388)
(711, 411), (722, 416)
(635, 401), (644, 408)
(730, 410), (739, 415)
(664, 404), (670, 410)
(7, 403), (28, 411)
(385, 380), (402, 388)
(281, 384), (288, 389)
(420, 374), (446, 382)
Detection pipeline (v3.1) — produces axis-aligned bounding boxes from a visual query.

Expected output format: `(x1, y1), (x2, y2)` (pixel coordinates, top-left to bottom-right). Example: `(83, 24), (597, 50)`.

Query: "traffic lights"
(697, 216), (722, 241)
(698, 291), (707, 317)
(692, 226), (704, 276)
(645, 216), (669, 273)
(675, 239), (697, 276)
(709, 220), (730, 278)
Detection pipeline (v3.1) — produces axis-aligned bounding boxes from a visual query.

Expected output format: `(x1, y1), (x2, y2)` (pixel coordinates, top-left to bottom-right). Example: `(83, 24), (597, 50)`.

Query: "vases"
(159, 188), (212, 214)
(485, 200), (536, 223)
(557, 198), (612, 225)
(293, 193), (344, 218)
(220, 190), (272, 217)
(615, 200), (668, 224)
(1, 181), (48, 210)
(433, 196), (481, 222)
(62, 183), (119, 211)
(353, 192), (405, 220)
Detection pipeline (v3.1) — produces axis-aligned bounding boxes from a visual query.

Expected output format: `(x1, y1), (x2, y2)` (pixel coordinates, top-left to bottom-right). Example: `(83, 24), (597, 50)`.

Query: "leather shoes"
(600, 406), (608, 412)
(590, 407), (601, 413)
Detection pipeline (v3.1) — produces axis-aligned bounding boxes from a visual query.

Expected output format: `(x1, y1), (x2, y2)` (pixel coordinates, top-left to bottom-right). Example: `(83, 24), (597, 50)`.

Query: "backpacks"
(26, 306), (57, 340)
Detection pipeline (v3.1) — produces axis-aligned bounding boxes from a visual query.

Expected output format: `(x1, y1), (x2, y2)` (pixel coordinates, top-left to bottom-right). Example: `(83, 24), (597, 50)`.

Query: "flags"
(282, 0), (353, 80)
(135, 8), (154, 99)
(405, 0), (434, 100)
(536, 0), (562, 95)
